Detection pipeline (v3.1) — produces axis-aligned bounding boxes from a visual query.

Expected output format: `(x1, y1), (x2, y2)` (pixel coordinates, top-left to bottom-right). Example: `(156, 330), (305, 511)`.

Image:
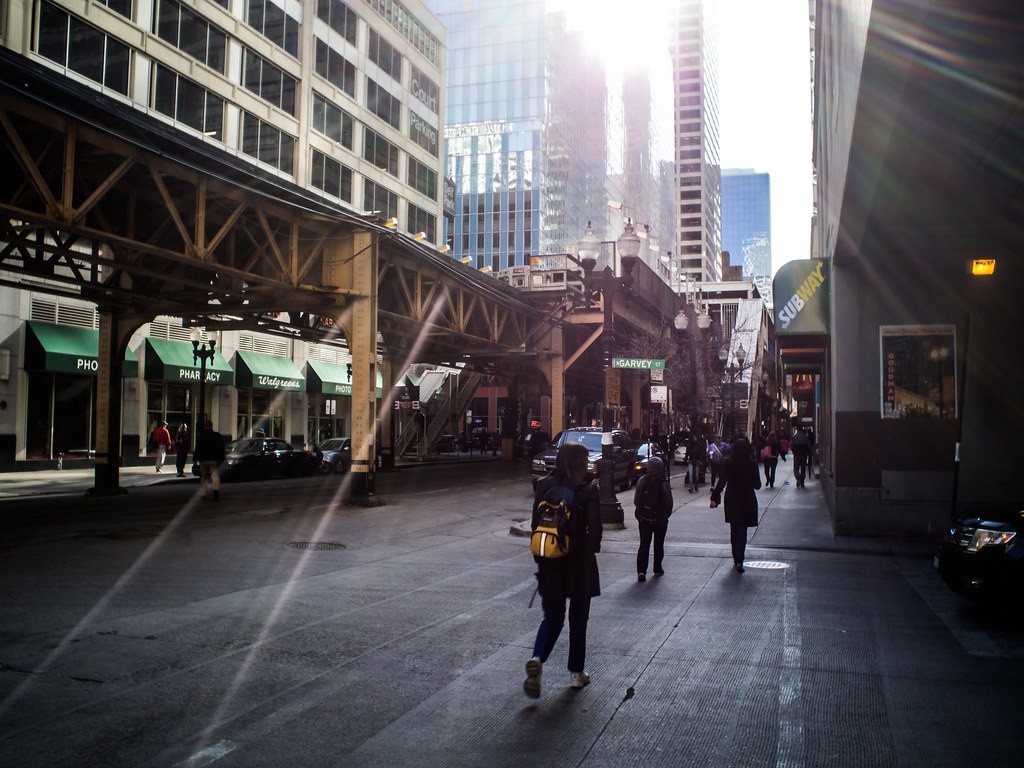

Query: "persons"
(151, 420), (172, 473)
(633, 457), (674, 581)
(705, 434), (733, 492)
(303, 443), (307, 451)
(172, 422), (191, 477)
(790, 425), (815, 488)
(311, 442), (318, 453)
(714, 436), (731, 444)
(779, 433), (789, 455)
(315, 447), (323, 462)
(729, 429), (755, 460)
(414, 408), (425, 443)
(760, 430), (786, 487)
(478, 429), (493, 454)
(683, 434), (703, 493)
(523, 442), (603, 699)
(192, 420), (226, 501)
(709, 441), (761, 574)
(255, 428), (265, 437)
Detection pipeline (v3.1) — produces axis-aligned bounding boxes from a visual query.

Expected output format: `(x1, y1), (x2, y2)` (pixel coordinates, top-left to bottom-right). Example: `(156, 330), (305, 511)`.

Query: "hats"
(162, 421), (168, 425)
(205, 421), (212, 427)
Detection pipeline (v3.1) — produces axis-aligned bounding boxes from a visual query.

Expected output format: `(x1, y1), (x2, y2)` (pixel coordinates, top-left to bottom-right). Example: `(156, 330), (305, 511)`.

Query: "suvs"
(530, 426), (636, 495)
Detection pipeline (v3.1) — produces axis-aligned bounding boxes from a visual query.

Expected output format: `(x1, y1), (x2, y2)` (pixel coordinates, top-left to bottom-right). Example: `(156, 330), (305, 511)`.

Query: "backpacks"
(530, 478), (581, 560)
(176, 431), (186, 448)
(635, 477), (666, 524)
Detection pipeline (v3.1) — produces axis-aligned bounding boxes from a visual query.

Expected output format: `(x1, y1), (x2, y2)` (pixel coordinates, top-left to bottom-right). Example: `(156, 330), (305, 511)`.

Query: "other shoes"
(654, 569), (664, 576)
(638, 572), (646, 582)
(735, 562), (745, 574)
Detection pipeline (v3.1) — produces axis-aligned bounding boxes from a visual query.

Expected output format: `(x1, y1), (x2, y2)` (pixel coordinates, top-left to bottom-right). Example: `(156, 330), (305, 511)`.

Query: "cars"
(192, 436), (318, 483)
(933, 505), (1024, 608)
(675, 437), (693, 464)
(311, 436), (381, 474)
(631, 439), (669, 480)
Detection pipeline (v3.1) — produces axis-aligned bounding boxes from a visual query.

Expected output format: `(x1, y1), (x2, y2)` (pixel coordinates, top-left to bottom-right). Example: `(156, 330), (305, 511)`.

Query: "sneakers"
(524, 656), (543, 698)
(570, 671), (590, 687)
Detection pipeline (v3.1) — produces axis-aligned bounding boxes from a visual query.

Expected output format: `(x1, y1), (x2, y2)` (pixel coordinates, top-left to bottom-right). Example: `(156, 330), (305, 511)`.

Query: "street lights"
(190, 328), (218, 468)
(671, 298), (714, 465)
(575, 215), (646, 530)
(718, 342), (748, 441)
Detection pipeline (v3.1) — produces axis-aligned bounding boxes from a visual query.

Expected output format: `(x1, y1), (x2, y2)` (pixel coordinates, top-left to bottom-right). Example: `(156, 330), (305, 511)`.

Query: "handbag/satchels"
(192, 464), (200, 476)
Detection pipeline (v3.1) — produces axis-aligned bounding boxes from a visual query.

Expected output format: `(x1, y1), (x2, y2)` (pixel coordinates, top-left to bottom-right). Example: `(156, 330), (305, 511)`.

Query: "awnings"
(773, 259), (828, 376)
(25, 320), (138, 379)
(235, 350), (306, 392)
(307, 361), (406, 399)
(405, 374), (446, 395)
(145, 336), (233, 386)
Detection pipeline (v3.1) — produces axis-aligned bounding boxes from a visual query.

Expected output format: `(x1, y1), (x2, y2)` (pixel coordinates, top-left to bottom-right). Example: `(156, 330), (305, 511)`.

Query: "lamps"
(439, 245), (450, 252)
(478, 266), (492, 273)
(458, 256), (473, 264)
(412, 231), (425, 240)
(385, 217), (397, 226)
